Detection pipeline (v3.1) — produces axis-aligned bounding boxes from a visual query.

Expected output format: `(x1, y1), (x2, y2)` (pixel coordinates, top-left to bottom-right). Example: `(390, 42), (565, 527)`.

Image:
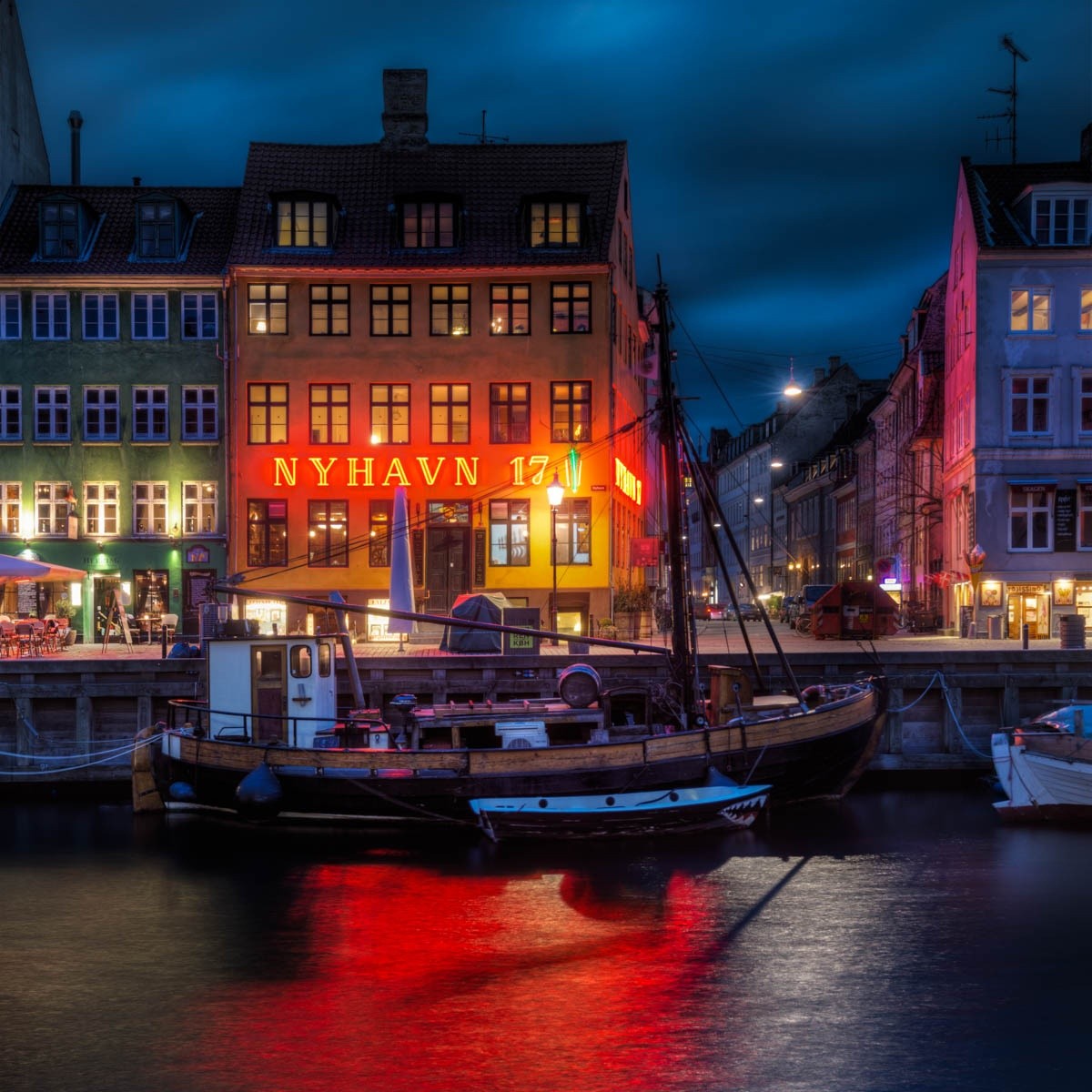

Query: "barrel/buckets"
(558, 663), (601, 708)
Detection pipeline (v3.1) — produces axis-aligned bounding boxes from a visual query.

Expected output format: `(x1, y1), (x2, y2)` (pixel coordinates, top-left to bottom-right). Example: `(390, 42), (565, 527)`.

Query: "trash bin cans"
(987, 615), (1004, 639)
(1060, 614), (1087, 648)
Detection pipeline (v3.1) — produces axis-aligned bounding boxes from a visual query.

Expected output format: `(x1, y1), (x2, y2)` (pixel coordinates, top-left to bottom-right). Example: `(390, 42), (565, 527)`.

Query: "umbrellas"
(387, 491), (419, 651)
(0, 555), (87, 616)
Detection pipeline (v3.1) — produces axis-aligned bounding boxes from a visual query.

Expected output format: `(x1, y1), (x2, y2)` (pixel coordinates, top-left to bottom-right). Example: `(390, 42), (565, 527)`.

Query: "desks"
(32, 627), (44, 655)
(135, 618), (161, 645)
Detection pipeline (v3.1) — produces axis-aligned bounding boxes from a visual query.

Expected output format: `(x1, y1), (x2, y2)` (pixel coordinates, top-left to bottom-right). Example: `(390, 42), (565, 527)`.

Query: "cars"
(779, 585), (836, 633)
(695, 603), (761, 622)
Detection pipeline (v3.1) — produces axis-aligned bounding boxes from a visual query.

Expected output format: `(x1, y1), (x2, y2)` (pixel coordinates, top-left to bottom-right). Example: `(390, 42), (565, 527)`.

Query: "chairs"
(0, 612), (68, 661)
(118, 614), (141, 647)
(158, 614), (178, 644)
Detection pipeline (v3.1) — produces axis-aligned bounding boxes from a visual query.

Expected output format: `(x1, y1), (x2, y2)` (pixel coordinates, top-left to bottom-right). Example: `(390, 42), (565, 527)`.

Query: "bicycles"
(796, 619), (814, 639)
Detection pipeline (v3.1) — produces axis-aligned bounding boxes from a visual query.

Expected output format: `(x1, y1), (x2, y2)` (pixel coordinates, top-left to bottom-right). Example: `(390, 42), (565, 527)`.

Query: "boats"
(141, 251), (889, 822)
(987, 703), (1092, 814)
(473, 771), (770, 837)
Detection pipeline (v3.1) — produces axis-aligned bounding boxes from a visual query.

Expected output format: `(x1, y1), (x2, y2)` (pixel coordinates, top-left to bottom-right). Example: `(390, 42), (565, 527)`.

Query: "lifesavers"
(798, 685), (833, 712)
